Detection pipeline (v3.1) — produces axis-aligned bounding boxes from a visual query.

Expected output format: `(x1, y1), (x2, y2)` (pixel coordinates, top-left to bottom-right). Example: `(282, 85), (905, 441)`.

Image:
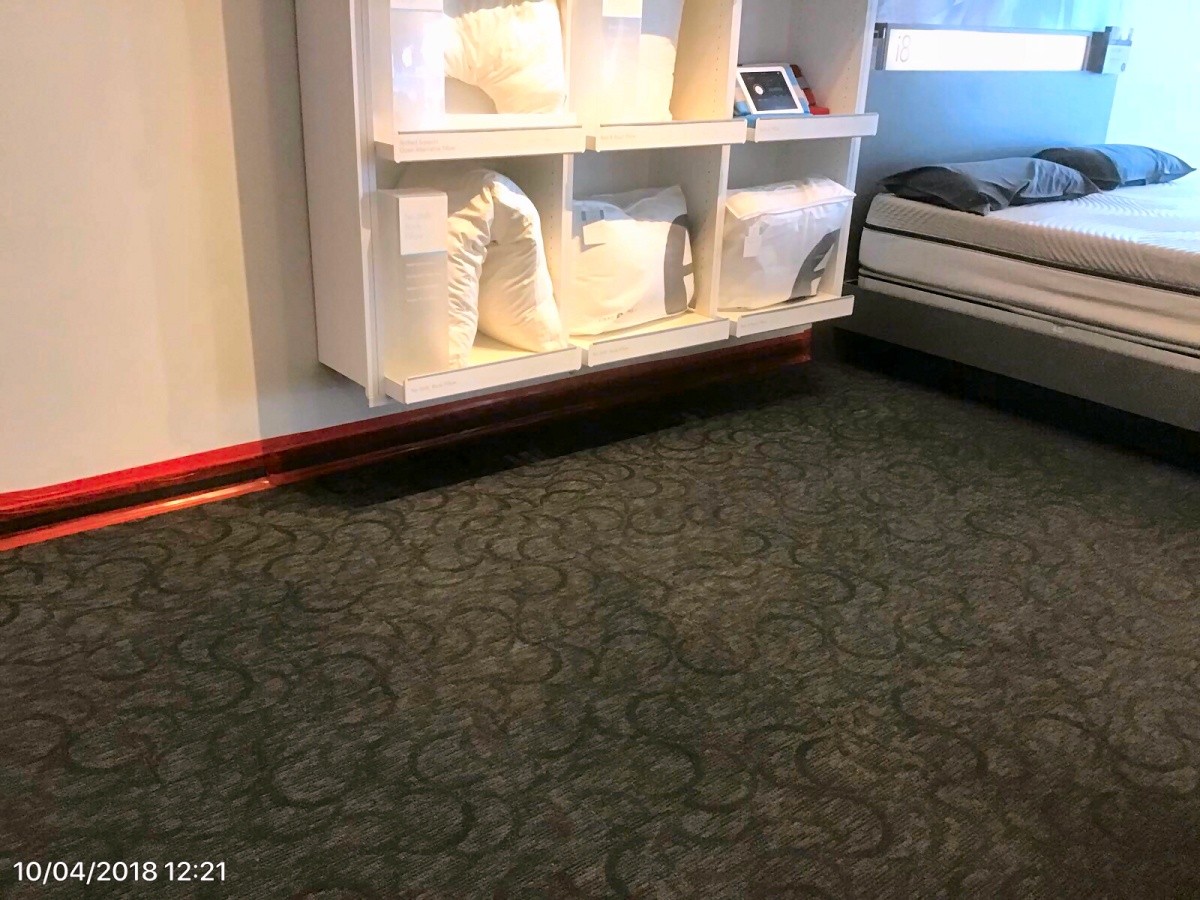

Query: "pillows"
(1032, 144), (1196, 192)
(877, 157), (1102, 215)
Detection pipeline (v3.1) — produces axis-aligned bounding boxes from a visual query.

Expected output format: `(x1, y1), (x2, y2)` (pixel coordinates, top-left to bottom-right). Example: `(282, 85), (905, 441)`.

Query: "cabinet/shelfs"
(292, 0), (877, 410)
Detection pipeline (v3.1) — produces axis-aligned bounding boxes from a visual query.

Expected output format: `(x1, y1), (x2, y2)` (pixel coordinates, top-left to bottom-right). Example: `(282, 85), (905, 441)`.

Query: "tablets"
(736, 65), (803, 114)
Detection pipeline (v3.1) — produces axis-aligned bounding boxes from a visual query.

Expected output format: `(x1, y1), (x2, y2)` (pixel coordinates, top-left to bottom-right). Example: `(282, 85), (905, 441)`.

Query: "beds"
(832, 142), (1200, 430)
